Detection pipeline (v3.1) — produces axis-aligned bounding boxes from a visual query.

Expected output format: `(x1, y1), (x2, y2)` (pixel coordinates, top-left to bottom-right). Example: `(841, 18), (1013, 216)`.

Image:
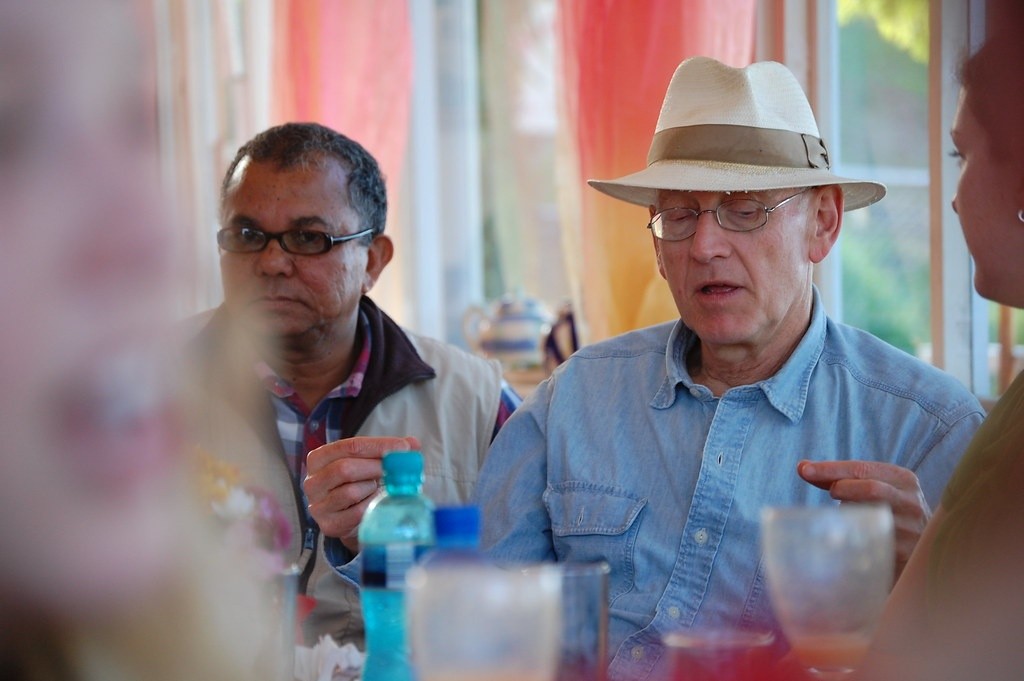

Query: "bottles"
(358, 452), (435, 681)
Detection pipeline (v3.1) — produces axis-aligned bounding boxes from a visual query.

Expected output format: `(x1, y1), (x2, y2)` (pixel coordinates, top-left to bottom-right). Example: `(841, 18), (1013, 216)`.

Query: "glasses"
(648, 190), (818, 241)
(217, 226), (378, 256)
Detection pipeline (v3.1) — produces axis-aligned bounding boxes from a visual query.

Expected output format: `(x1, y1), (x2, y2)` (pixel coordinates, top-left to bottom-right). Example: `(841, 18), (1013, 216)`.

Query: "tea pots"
(461, 292), (558, 385)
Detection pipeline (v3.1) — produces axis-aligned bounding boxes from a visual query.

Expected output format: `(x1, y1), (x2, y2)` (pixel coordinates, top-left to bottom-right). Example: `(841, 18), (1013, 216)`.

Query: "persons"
(163, 122), (525, 653)
(856, 0), (1024, 681)
(305, 57), (989, 681)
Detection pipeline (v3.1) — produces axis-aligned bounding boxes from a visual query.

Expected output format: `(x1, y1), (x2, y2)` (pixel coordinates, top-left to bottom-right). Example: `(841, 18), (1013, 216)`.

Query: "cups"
(546, 561), (611, 681)
(410, 566), (561, 681)
(762, 504), (897, 681)
(666, 631), (775, 681)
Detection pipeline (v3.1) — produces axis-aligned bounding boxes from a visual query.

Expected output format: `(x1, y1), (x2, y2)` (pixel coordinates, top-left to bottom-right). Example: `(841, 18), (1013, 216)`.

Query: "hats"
(588, 56), (886, 212)
